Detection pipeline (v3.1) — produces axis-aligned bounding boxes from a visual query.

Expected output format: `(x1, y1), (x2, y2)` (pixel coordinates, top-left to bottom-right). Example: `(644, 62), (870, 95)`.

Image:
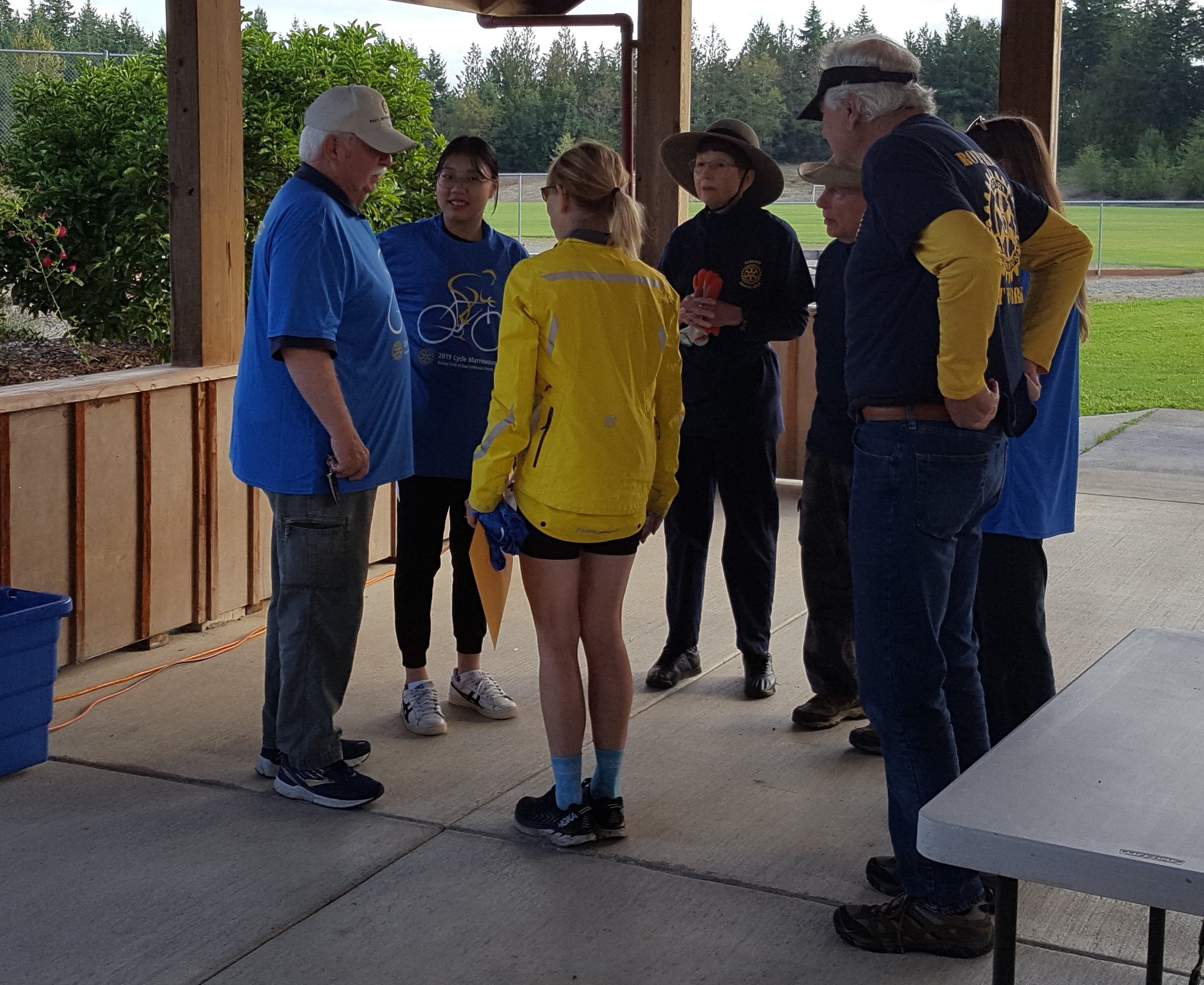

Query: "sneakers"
(399, 680), (447, 737)
(256, 739), (369, 776)
(513, 783), (597, 847)
(741, 654), (777, 696)
(582, 776), (630, 839)
(274, 755), (384, 808)
(865, 856), (996, 914)
(792, 693), (867, 729)
(646, 640), (703, 689)
(832, 891), (994, 959)
(847, 724), (879, 755)
(448, 666), (518, 719)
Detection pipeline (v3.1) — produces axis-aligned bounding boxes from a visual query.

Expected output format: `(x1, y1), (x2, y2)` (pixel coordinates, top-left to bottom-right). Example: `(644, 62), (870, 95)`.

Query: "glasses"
(434, 171), (490, 189)
(964, 114), (1004, 158)
(540, 184), (567, 202)
(688, 159), (743, 173)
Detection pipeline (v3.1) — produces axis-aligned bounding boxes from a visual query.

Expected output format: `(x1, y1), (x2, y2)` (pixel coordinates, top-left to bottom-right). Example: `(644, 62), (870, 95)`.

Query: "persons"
(793, 154), (890, 758)
(373, 133), (521, 736)
(642, 124), (809, 699)
(232, 84), (408, 810)
(961, 112), (1090, 743)
(461, 139), (683, 850)
(821, 35), (1092, 962)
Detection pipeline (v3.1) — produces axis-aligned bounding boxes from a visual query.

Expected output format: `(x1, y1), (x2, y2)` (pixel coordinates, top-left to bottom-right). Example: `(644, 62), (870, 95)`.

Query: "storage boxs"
(0, 585), (73, 778)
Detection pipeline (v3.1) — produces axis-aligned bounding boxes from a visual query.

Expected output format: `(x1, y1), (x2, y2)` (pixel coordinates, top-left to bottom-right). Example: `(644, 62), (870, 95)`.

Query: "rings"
(698, 304), (703, 309)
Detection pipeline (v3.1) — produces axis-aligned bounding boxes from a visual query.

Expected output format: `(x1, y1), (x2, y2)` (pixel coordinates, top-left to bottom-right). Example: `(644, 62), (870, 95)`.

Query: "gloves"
(679, 269), (723, 346)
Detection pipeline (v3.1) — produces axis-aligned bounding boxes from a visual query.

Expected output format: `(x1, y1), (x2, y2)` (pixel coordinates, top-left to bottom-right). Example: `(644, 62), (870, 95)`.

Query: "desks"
(917, 626), (1204, 985)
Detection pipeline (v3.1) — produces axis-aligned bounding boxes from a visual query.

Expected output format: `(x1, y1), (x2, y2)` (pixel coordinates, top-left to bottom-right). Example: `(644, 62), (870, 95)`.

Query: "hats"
(795, 65), (918, 123)
(659, 119), (784, 208)
(797, 154), (862, 187)
(304, 84), (417, 153)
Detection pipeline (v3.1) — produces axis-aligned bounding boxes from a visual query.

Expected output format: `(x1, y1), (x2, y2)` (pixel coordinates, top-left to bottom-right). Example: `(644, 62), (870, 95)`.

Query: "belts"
(860, 406), (950, 423)
